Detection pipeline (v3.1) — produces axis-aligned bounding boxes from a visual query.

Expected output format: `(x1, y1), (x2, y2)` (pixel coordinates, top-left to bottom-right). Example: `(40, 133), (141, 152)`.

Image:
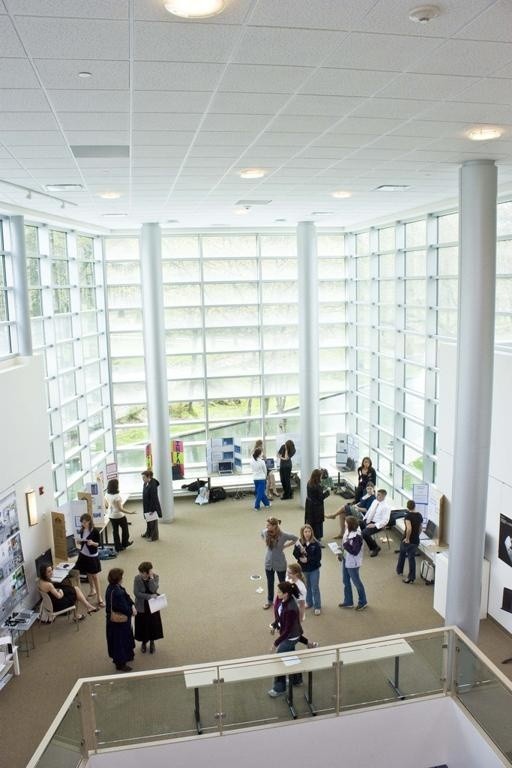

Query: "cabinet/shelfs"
(432, 547), (491, 620)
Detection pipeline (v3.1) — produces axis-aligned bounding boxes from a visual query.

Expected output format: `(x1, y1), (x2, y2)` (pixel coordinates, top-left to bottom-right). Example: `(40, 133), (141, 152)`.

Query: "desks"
(2, 612), (41, 656)
(36, 492), (129, 590)
(172, 467), (292, 502)
(0, 644), (21, 691)
(182, 633), (414, 734)
(344, 471), (450, 566)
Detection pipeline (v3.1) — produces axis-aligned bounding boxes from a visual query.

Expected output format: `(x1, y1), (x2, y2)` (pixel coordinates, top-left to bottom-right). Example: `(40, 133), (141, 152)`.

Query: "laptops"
(262, 458), (276, 471)
(419, 519), (436, 540)
(66, 534), (81, 556)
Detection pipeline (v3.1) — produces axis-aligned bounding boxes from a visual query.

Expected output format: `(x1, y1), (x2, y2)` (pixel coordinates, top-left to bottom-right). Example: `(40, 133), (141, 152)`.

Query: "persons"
(104, 479), (137, 552)
(35, 563), (100, 622)
(105, 568), (139, 671)
(140, 471), (162, 541)
(133, 562), (159, 654)
(73, 513), (105, 607)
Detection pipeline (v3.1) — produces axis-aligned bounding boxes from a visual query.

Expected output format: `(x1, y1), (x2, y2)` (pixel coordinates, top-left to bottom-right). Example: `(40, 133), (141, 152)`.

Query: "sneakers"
(286, 679), (304, 688)
(140, 643), (156, 655)
(267, 689), (288, 697)
(303, 603), (309, 608)
(112, 659), (134, 673)
(369, 547), (382, 559)
(339, 601), (353, 610)
(355, 600), (368, 613)
(142, 533), (160, 542)
(314, 609), (322, 615)
(250, 490), (294, 510)
(332, 534), (342, 539)
(115, 540), (135, 552)
(262, 601), (272, 608)
(324, 513), (336, 520)
(306, 641), (320, 649)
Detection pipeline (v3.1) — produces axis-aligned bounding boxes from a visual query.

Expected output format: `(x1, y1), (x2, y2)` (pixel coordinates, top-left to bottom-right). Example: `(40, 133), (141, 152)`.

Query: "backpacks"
(181, 481), (226, 506)
(341, 535), (363, 556)
(358, 469), (370, 487)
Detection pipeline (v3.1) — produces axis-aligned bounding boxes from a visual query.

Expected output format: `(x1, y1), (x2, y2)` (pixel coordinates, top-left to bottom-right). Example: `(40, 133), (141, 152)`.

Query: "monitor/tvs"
(35, 548), (53, 577)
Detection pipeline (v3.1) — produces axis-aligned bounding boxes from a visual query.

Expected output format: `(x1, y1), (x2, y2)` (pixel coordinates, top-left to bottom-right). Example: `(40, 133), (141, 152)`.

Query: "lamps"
(24, 488), (40, 526)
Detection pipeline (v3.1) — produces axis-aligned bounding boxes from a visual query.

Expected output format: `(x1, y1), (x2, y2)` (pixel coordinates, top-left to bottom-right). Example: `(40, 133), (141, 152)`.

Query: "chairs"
(39, 591), (79, 641)
(372, 511), (395, 550)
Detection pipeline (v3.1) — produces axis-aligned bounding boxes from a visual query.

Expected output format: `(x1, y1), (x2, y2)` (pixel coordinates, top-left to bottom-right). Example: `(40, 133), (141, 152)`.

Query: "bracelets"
(150, 577), (153, 580)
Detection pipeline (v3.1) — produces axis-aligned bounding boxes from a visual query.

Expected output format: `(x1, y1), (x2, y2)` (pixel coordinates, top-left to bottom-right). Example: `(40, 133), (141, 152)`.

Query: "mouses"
(63, 564), (69, 568)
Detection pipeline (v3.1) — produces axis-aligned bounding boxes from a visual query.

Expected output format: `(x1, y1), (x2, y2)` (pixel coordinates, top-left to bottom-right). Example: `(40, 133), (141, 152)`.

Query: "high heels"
(87, 607), (102, 616)
(74, 614), (86, 623)
(403, 576), (414, 585)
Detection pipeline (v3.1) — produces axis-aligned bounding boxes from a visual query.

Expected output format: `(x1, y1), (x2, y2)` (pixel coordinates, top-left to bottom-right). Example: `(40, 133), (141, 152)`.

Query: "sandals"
(87, 590), (97, 598)
(99, 599), (105, 607)
(109, 610), (129, 623)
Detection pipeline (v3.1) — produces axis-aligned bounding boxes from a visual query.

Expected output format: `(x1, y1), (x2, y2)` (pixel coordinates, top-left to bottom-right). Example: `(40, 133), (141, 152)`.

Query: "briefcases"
(419, 561), (437, 586)
